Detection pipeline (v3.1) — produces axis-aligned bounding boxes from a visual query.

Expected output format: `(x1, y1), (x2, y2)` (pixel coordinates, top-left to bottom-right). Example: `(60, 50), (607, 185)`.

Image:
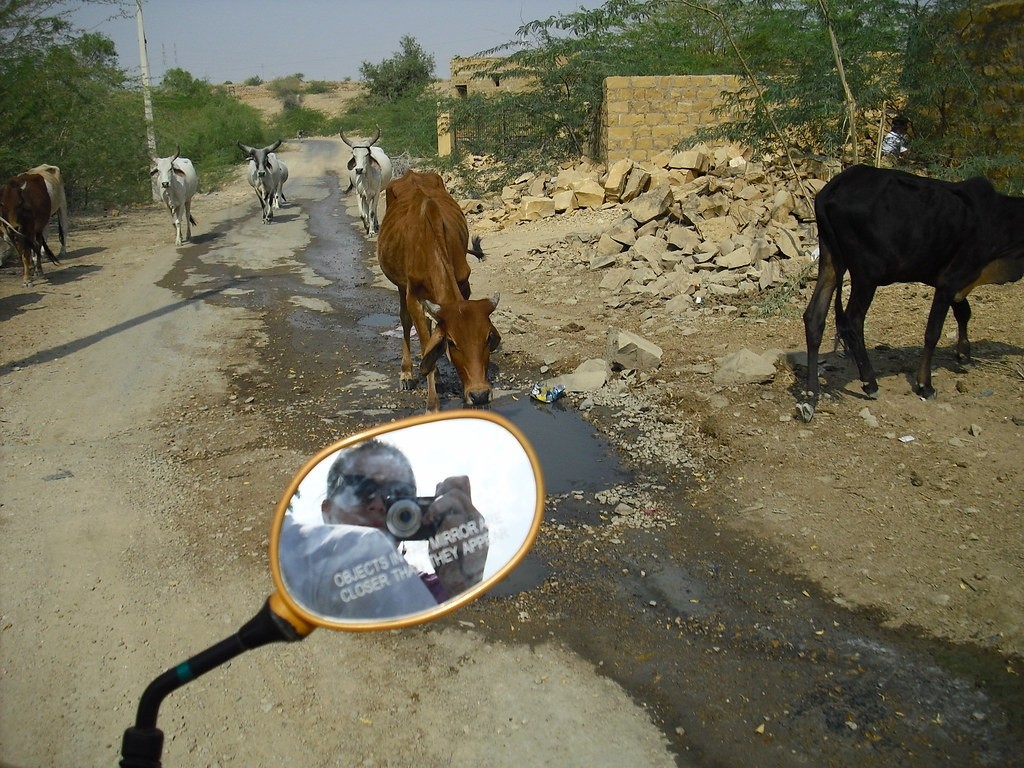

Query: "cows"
(237, 139), (289, 226)
(146, 143), (198, 246)
(0, 163), (70, 288)
(794, 163), (1024, 424)
(339, 123), (393, 239)
(376, 169), (502, 414)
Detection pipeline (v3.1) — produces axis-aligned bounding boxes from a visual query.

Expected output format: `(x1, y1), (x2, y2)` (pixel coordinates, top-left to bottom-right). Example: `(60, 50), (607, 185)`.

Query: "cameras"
(385, 495), (444, 541)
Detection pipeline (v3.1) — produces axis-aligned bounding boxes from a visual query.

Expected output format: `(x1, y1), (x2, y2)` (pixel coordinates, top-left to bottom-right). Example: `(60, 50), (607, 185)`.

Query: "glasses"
(328, 474), (417, 508)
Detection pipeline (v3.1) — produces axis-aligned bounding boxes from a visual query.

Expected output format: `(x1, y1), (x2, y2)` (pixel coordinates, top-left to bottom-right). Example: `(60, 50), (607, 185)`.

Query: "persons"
(321, 437), (490, 606)
(277, 485), (439, 621)
